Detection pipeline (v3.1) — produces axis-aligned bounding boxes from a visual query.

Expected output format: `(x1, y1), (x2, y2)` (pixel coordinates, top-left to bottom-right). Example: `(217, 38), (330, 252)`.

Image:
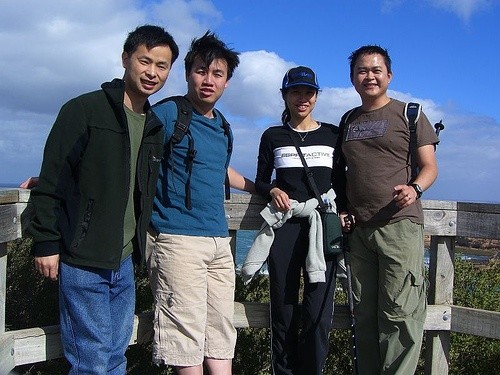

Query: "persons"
(254, 66), (356, 375)
(30, 25), (179, 375)
(21, 30), (260, 375)
(338, 45), (441, 375)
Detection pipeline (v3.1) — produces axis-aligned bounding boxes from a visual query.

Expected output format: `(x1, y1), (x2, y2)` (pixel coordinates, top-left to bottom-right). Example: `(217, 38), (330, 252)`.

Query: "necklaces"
(293, 120), (312, 142)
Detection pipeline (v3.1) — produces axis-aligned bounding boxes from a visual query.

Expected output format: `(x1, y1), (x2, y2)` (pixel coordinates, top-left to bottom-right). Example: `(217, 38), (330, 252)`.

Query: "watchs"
(410, 183), (423, 200)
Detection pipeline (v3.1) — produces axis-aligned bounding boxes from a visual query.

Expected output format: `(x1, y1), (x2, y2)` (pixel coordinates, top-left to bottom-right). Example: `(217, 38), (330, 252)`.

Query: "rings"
(407, 195), (411, 199)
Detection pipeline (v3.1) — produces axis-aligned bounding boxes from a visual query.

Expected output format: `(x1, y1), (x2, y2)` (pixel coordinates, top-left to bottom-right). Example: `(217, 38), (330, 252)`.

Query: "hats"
(282, 67), (320, 89)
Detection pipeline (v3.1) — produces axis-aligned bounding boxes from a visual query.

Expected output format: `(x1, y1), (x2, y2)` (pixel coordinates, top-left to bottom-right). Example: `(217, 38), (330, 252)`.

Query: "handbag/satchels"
(325, 212), (343, 255)
